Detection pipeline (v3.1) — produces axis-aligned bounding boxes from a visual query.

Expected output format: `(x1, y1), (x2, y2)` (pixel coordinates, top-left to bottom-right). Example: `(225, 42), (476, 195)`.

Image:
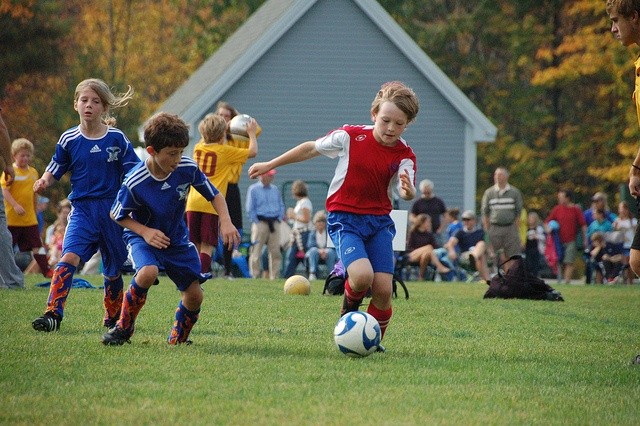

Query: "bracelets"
(632, 164), (640, 170)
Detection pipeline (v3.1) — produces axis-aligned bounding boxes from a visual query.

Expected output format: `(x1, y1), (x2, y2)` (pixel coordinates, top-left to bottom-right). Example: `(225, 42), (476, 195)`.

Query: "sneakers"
(102, 321), (133, 346)
(608, 276), (620, 285)
(33, 312), (62, 332)
(308, 273), (317, 281)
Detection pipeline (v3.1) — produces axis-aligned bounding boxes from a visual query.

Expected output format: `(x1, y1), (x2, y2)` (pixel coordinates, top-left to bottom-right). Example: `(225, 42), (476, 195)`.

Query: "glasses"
(462, 217), (474, 222)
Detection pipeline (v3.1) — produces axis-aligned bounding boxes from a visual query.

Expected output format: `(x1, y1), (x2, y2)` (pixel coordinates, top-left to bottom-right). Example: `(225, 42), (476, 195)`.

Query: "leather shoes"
(436, 268), (450, 274)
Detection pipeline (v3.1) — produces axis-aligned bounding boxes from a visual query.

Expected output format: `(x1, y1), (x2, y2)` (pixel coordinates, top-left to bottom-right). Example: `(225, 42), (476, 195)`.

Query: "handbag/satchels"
(322, 271), (409, 300)
(483, 254), (565, 302)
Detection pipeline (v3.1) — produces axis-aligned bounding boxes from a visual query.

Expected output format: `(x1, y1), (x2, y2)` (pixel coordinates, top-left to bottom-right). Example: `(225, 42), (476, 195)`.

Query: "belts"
(490, 222), (513, 226)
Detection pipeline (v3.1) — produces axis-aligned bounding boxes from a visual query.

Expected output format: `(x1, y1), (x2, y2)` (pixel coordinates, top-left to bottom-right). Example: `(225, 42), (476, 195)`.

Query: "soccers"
(283, 274), (311, 295)
(334, 311), (382, 358)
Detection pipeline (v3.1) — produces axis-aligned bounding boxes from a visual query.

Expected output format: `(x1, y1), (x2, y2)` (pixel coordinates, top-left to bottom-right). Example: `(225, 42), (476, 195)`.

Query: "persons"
(603, 1), (640, 367)
(546, 190), (587, 283)
(285, 179), (314, 280)
(406, 214), (447, 281)
(48, 225), (66, 264)
(1, 138), (53, 277)
(527, 211), (553, 276)
(584, 192), (619, 227)
(481, 167), (523, 265)
(590, 233), (625, 285)
(31, 77), (141, 332)
(36, 190), (51, 240)
(586, 210), (614, 248)
(1, 114), (24, 290)
(443, 211), (491, 283)
(443, 208), (463, 253)
(23, 200), (85, 275)
(102, 113), (241, 346)
(306, 209), (340, 280)
(408, 179), (448, 237)
(611, 200), (639, 284)
(186, 113), (258, 274)
(214, 100), (262, 279)
(248, 80), (417, 352)
(246, 167), (285, 279)
